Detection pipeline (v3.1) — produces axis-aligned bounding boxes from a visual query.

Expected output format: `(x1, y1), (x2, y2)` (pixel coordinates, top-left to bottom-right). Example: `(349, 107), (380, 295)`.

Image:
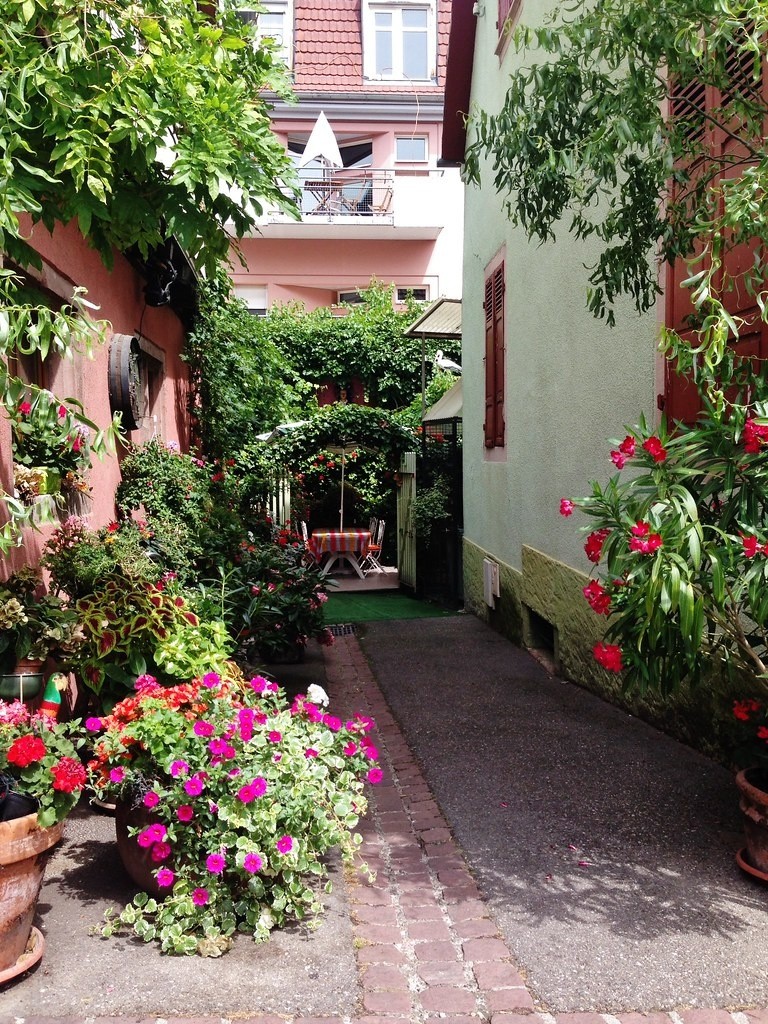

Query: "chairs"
(358, 516), (387, 579)
(294, 519), (320, 570)
(366, 187), (394, 217)
(341, 177), (371, 215)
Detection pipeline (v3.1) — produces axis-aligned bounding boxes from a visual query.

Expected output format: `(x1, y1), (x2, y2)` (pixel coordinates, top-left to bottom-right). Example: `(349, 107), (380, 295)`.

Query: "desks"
(318, 526), (372, 582)
(304, 179), (345, 217)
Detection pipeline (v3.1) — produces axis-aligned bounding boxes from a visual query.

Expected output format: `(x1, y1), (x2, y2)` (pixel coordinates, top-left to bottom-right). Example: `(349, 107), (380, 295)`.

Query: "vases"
(3, 655), (48, 706)
(0, 806), (64, 987)
(116, 796), (169, 893)
(733, 769), (768, 882)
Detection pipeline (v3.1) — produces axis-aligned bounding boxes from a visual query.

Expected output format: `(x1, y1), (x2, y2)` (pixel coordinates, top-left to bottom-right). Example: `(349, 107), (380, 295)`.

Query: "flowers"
(558, 363), (767, 795)
(0, 399), (386, 960)
